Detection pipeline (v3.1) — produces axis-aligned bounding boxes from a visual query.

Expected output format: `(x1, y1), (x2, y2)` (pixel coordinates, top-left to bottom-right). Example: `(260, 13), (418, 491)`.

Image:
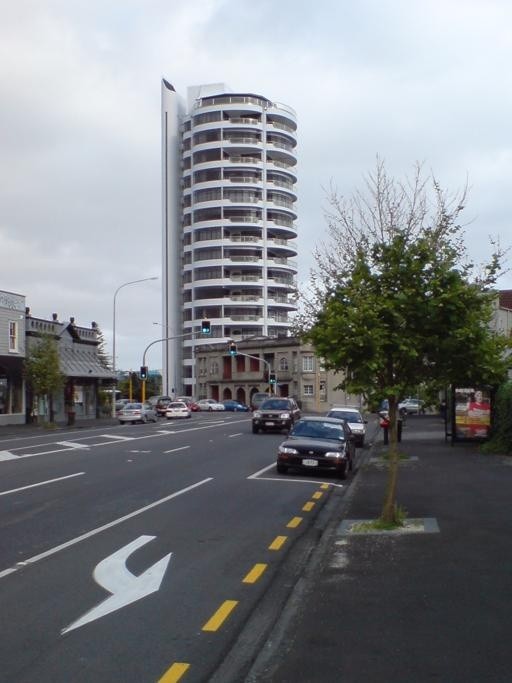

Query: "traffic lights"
(270, 373), (277, 384)
(201, 320), (211, 335)
(229, 343), (238, 357)
(139, 367), (148, 381)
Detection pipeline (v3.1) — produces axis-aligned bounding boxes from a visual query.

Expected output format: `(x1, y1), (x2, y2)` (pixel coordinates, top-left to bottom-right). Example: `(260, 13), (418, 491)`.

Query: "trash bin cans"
(67, 410), (74, 425)
(381, 420), (403, 445)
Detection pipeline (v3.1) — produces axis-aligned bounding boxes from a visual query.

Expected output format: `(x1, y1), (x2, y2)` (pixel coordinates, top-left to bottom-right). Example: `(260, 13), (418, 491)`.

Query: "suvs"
(148, 395), (172, 416)
(397, 398), (425, 416)
(252, 396), (301, 435)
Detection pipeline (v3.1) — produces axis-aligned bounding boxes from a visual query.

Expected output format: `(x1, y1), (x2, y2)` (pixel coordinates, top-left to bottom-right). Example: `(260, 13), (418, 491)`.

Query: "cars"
(222, 399), (249, 413)
(116, 399), (138, 410)
(118, 403), (158, 425)
(323, 407), (369, 446)
(165, 401), (191, 419)
(175, 396), (201, 412)
(194, 399), (225, 412)
(275, 416), (358, 480)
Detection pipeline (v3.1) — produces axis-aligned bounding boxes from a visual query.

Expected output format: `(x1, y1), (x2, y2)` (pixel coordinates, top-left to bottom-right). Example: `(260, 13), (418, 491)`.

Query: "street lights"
(152, 321), (177, 400)
(111, 276), (158, 421)
